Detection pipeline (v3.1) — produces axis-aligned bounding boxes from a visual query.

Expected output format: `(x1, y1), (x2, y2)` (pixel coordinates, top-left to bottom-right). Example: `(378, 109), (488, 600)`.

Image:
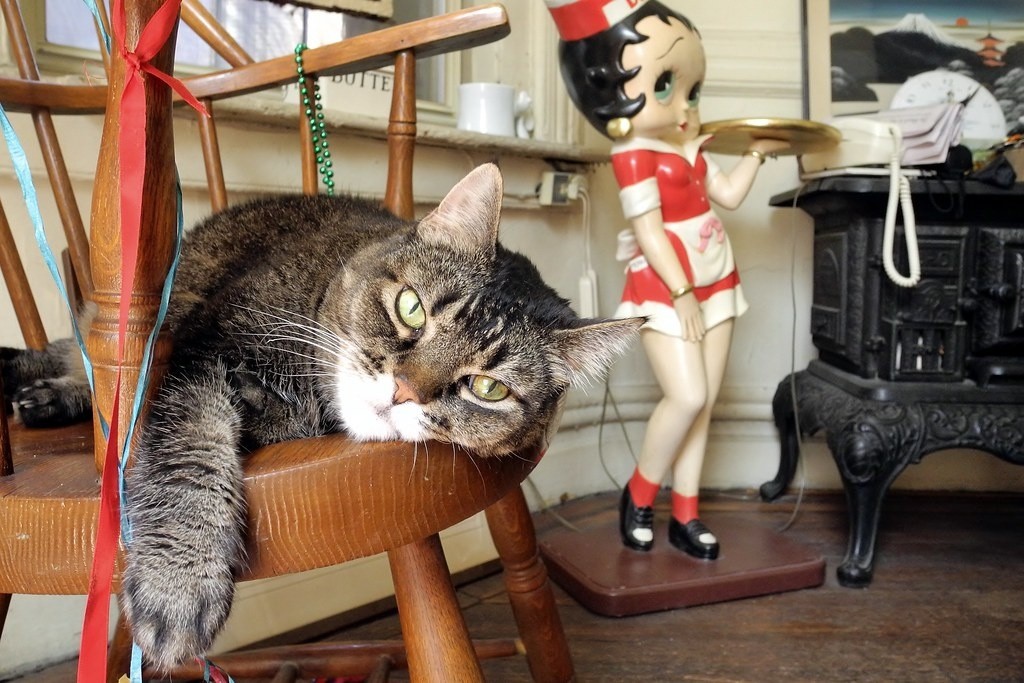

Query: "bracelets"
(668, 284), (692, 301)
(742, 149), (766, 165)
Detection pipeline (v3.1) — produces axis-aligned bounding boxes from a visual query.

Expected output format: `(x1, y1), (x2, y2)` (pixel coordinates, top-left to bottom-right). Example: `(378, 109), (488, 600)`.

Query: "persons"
(542, 1), (792, 561)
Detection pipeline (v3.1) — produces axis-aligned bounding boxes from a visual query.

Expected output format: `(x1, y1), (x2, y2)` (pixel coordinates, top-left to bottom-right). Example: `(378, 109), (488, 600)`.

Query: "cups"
(456, 81), (518, 138)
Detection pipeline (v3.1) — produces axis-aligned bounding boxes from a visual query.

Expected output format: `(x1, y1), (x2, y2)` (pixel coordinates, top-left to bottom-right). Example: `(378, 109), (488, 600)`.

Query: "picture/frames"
(803, 0), (1024, 176)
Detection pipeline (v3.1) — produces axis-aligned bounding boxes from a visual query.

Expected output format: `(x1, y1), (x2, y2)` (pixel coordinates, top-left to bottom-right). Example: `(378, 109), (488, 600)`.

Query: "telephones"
(799, 114), (906, 169)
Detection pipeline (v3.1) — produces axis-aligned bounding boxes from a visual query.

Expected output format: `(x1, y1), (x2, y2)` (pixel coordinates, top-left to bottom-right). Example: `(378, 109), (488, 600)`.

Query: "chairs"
(1, 1), (576, 682)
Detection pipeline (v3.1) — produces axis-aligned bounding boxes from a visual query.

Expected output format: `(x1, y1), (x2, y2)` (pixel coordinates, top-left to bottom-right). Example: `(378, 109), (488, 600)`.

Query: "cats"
(1, 163), (654, 673)
